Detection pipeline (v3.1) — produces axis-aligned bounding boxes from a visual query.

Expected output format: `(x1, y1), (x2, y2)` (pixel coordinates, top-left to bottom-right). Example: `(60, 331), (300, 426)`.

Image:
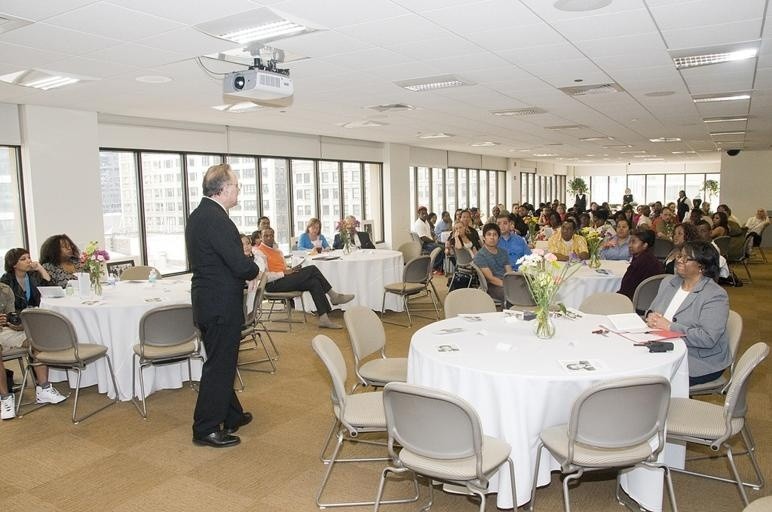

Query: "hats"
(418, 206), (427, 212)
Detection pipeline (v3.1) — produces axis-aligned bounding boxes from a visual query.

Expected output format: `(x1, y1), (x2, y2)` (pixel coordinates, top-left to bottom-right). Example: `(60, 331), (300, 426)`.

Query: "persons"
(233, 213), (379, 336)
(0, 246), (58, 327)
(184, 164), (260, 448)
(39, 234), (91, 290)
(641, 240), (735, 387)
(615, 229), (664, 315)
(1, 282), (69, 420)
(412, 188), (770, 310)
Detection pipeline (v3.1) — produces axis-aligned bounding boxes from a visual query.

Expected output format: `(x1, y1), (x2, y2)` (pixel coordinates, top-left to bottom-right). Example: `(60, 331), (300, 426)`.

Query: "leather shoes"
(193, 431), (241, 447)
(330, 294), (355, 305)
(318, 321), (344, 329)
(224, 412), (252, 433)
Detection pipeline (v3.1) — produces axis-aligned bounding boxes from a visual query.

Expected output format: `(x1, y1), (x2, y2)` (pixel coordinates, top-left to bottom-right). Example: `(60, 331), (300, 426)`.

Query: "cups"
(315, 240), (323, 254)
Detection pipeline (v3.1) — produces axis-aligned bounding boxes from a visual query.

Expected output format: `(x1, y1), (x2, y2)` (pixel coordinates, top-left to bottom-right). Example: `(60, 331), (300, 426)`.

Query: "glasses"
(675, 255), (696, 263)
(228, 183), (243, 189)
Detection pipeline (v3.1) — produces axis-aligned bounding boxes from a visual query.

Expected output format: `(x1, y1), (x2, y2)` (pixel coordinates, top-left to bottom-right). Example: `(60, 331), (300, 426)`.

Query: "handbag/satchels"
(447, 273), (471, 291)
(718, 272), (742, 287)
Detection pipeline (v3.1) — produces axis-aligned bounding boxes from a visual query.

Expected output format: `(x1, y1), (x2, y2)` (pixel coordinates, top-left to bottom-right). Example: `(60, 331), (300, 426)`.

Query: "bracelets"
(39, 266), (45, 274)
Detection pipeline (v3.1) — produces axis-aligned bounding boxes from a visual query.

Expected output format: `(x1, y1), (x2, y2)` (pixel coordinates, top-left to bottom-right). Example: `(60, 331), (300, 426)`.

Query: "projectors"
(222, 71), (294, 100)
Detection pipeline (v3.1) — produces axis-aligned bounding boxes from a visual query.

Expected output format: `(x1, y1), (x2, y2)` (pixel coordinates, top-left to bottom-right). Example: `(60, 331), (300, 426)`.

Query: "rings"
(653, 326), (656, 329)
(653, 317), (657, 322)
(653, 322), (656, 326)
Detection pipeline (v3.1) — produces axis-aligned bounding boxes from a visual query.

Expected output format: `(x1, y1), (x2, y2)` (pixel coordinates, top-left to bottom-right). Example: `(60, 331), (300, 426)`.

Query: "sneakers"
(432, 270), (444, 275)
(36, 384), (67, 403)
(1, 393), (16, 419)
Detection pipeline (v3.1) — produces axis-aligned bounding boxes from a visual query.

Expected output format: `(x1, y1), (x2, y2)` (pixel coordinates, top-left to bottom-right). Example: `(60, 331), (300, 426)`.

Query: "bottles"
(108, 272), (115, 287)
(148, 268), (157, 288)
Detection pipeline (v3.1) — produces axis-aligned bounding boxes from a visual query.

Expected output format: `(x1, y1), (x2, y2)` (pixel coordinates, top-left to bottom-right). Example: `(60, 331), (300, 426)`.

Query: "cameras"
(6, 313), (22, 326)
